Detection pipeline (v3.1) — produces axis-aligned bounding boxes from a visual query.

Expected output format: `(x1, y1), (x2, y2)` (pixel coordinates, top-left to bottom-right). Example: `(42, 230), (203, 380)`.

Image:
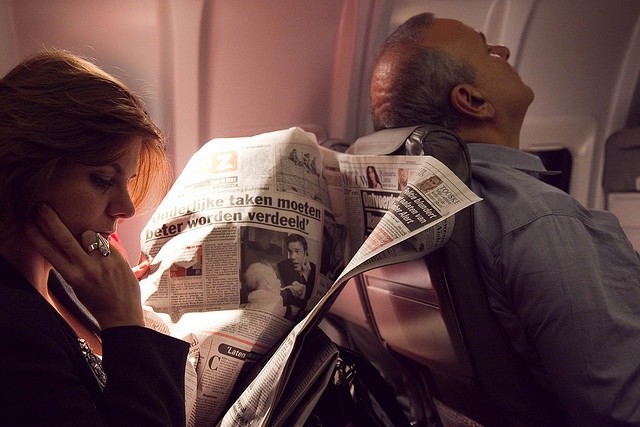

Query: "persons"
(398, 168), (407, 190)
(327, 238), (345, 281)
(417, 175), (443, 193)
(279, 233), (317, 322)
(288, 148), (318, 176)
(244, 260), (288, 318)
(0, 47), (190, 423)
(369, 12), (640, 426)
(366, 165), (382, 188)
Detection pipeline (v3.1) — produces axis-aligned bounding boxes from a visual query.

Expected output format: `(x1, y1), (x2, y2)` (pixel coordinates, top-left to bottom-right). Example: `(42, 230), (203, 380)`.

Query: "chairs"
(341, 125), (568, 427)
(314, 136), (424, 425)
